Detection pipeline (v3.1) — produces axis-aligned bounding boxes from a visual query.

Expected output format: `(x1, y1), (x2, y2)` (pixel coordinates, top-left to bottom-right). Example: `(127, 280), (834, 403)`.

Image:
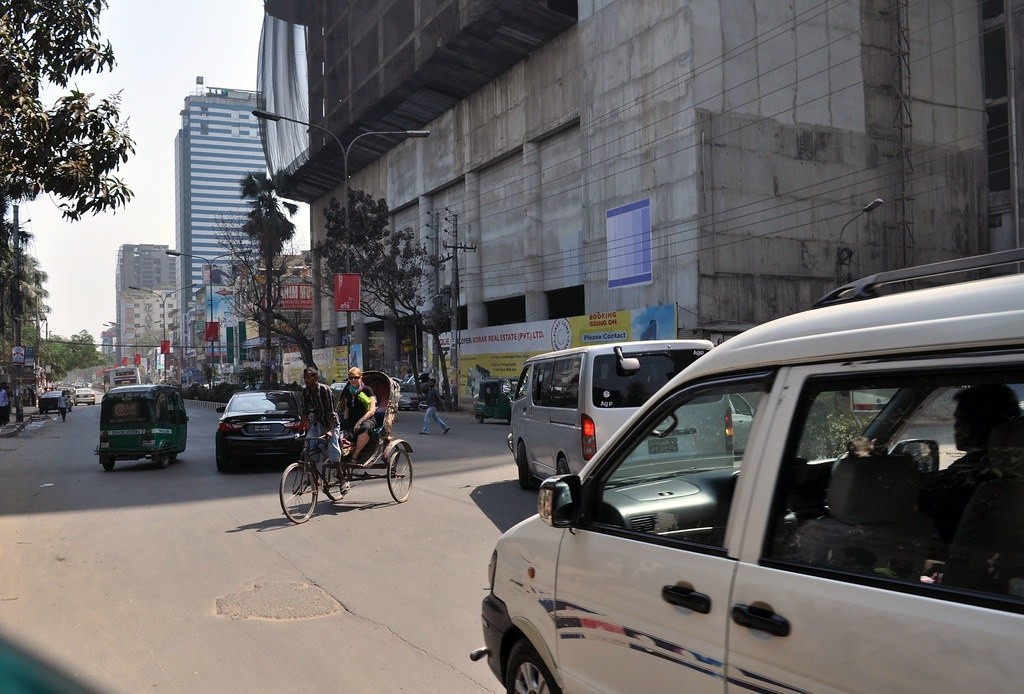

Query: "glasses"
(349, 376), (361, 380)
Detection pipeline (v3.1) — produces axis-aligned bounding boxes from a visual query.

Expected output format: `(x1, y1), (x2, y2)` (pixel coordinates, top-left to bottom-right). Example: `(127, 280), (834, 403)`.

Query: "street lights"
(165, 249), (254, 389)
(838, 197), (886, 288)
(251, 107), (431, 377)
(102, 320), (162, 366)
(128, 283), (198, 384)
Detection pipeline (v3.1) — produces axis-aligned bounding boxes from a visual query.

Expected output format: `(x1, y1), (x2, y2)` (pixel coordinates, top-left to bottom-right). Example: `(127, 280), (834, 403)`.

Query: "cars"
(214, 389), (309, 475)
(329, 377), (427, 411)
(37, 387), (95, 414)
(726, 392), (756, 455)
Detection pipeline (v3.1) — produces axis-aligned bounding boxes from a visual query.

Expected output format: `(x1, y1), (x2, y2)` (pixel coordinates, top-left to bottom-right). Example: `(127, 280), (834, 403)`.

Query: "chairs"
(951, 416), (1024, 568)
(240, 403), (253, 409)
(336, 369), (401, 449)
(783, 452), (929, 581)
(277, 402), (289, 410)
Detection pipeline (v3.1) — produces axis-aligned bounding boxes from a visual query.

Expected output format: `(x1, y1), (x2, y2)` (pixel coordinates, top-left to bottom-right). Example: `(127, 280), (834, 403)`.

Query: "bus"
(102, 366), (142, 393)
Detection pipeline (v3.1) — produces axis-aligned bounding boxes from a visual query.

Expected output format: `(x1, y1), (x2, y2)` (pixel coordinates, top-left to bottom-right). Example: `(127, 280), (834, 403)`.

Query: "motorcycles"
(472, 377), (520, 425)
(93, 384), (189, 471)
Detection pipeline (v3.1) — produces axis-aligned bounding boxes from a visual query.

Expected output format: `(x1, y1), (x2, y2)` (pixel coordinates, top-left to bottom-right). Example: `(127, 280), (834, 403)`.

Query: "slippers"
(303, 480), (321, 486)
(339, 481), (351, 496)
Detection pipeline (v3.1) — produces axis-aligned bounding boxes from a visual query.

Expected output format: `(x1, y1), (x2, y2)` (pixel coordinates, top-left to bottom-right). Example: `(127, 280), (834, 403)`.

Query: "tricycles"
(278, 369), (414, 524)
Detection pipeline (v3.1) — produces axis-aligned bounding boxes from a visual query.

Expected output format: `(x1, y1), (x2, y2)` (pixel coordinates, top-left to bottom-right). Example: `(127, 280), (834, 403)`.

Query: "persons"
(936, 385), (1024, 511)
(0, 382), (57, 426)
(58, 390), (68, 422)
(340, 367), (376, 463)
(294, 367), (350, 496)
(419, 379), (450, 434)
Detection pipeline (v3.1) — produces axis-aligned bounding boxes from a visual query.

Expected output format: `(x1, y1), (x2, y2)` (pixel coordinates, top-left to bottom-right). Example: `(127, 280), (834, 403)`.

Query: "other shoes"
(418, 432), (430, 435)
(443, 428), (450, 434)
(351, 456), (358, 464)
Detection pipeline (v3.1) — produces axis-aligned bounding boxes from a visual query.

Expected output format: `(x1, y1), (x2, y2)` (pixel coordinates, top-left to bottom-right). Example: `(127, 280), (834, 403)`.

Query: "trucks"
(469, 271), (1023, 694)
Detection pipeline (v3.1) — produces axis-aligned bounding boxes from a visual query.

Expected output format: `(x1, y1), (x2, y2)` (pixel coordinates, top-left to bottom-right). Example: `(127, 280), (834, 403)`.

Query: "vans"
(501, 339), (735, 490)
